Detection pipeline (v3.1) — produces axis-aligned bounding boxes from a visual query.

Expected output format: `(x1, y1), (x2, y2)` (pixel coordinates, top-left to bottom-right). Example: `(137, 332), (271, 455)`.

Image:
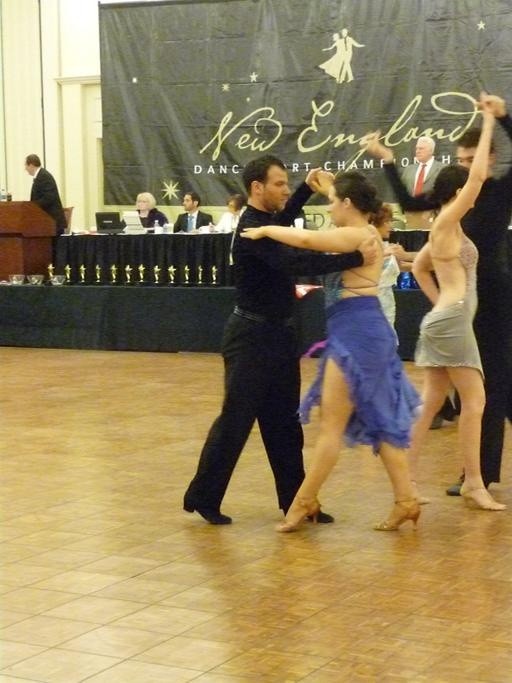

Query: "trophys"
(46, 263), (221, 286)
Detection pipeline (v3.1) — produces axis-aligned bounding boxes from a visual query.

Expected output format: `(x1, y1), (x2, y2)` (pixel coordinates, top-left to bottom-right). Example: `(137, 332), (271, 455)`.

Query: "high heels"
(373, 498), (421, 531)
(276, 493), (321, 533)
(460, 485), (508, 510)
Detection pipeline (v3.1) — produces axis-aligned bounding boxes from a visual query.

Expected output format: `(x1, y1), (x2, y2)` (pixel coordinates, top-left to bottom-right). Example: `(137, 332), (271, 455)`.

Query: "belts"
(233, 305), (298, 327)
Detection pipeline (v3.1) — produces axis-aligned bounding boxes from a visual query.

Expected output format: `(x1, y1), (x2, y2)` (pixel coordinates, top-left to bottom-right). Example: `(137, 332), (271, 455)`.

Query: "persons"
(408, 92), (508, 511)
(135, 192), (168, 234)
(182, 155), (379, 525)
(208, 193), (246, 232)
(364, 95), (512, 498)
(174, 192), (214, 232)
(239, 171), (427, 533)
(23, 155), (68, 260)
(401, 136), (447, 199)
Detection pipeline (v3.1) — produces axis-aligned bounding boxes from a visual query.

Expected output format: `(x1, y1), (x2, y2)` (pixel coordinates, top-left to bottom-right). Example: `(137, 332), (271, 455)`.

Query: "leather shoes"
(283, 508), (335, 522)
(447, 485), (463, 496)
(182, 497), (232, 525)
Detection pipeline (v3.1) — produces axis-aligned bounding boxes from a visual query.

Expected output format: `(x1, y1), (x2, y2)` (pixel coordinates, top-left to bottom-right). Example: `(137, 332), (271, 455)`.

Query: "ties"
(415, 164), (426, 198)
(187, 215), (193, 232)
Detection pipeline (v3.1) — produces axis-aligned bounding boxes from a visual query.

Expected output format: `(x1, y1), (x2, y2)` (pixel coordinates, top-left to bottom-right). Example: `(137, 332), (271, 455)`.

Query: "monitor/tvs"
(95, 212), (121, 234)
